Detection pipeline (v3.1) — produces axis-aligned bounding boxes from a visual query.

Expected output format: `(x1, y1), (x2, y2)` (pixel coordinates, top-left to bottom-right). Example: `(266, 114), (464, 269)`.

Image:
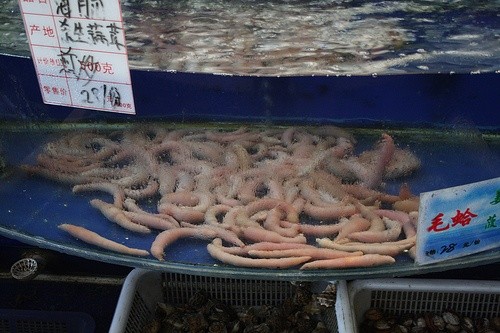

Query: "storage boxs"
(349, 278), (499, 333)
(0, 308), (96, 333)
(0, 120), (499, 282)
(107, 268), (356, 333)
(0, 0), (500, 134)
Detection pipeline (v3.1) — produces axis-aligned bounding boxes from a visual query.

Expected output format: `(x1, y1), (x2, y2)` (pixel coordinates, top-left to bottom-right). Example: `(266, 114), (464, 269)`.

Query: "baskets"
(348, 279), (500, 332)
(108, 268), (355, 333)
